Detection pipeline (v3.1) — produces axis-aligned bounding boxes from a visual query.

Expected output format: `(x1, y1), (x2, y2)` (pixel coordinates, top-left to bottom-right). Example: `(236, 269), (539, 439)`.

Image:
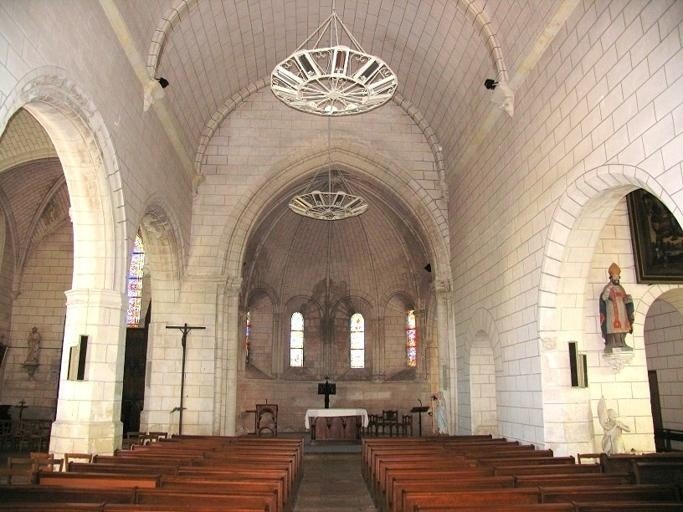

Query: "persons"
(603, 409), (630, 455)
(600, 262), (635, 354)
(27, 327), (40, 363)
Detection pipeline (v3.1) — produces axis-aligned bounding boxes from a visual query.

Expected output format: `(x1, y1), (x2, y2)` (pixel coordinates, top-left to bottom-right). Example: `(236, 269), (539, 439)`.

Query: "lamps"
(267, 0), (400, 118)
(285, 118), (371, 223)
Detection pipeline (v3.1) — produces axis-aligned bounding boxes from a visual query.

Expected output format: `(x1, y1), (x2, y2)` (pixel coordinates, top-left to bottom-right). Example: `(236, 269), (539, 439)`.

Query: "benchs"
(360, 432), (682, 512)
(0, 415), (305, 511)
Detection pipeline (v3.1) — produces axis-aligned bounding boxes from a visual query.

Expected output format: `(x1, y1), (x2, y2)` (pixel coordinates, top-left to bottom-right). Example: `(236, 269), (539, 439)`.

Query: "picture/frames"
(626, 188), (682, 287)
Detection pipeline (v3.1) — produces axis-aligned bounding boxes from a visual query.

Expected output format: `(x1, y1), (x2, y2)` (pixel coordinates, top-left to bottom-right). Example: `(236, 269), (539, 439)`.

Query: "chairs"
(367, 409), (413, 436)
